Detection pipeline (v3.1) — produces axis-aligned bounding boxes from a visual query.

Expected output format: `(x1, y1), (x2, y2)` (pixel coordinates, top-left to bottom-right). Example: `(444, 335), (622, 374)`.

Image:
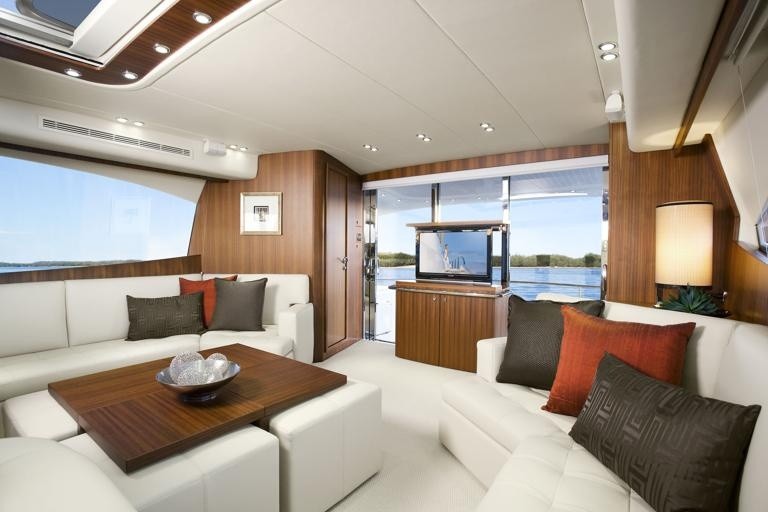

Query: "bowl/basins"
(155, 359), (242, 405)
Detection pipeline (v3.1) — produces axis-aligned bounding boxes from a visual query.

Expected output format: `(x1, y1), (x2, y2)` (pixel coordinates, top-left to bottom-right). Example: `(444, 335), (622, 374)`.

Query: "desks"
(4, 343), (387, 512)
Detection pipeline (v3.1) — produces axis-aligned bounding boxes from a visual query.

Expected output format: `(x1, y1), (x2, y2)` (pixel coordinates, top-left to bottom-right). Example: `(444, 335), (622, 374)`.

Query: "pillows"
(569, 350), (762, 512)
(436, 295), (768, 512)
(123, 291), (203, 342)
(540, 303), (695, 416)
(209, 277), (269, 331)
(178, 275), (239, 329)
(496, 294), (604, 390)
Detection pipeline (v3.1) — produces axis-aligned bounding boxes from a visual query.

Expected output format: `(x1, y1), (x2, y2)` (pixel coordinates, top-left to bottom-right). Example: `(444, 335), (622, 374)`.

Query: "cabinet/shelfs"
(395, 281), (508, 373)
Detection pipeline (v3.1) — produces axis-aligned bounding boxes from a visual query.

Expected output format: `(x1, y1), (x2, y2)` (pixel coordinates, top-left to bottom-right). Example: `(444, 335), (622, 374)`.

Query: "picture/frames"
(240, 191), (283, 236)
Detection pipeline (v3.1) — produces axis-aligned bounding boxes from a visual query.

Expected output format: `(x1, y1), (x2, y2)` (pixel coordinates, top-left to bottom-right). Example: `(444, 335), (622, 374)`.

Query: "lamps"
(653, 201), (715, 303)
(604, 92), (623, 113)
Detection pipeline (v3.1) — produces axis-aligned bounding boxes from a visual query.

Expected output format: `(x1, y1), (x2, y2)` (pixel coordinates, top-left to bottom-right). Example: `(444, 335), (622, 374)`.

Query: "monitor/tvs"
(416, 229), (492, 284)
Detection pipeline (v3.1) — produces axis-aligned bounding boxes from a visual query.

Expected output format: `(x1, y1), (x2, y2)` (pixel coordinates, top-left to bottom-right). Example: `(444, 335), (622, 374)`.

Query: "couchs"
(1, 271), (315, 405)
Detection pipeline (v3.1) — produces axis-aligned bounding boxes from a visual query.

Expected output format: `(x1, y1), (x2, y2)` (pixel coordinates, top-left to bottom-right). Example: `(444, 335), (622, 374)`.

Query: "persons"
(443, 244), (450, 272)
